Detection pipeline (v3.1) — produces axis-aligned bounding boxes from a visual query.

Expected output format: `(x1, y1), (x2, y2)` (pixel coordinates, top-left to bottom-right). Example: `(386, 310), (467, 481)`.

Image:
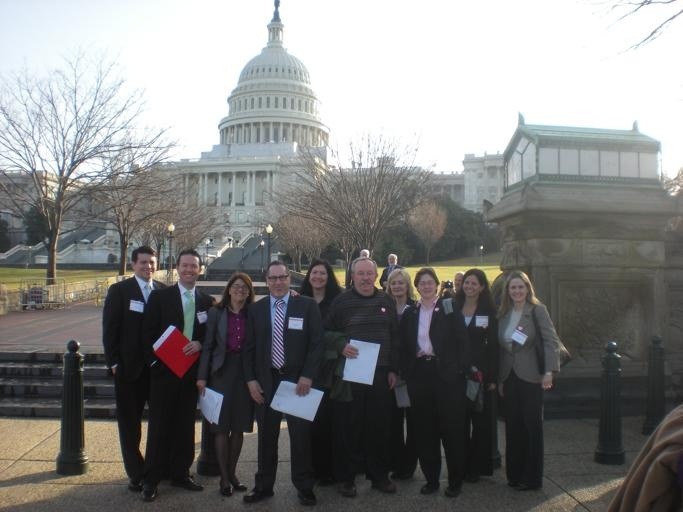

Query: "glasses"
(268, 274), (289, 282)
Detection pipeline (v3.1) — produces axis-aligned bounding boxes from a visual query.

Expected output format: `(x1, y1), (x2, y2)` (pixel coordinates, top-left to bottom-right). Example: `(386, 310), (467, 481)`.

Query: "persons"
(104, 247), (561, 504)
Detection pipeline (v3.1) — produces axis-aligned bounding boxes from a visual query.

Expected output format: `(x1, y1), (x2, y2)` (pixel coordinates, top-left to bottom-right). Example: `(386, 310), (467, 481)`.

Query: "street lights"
(259, 240), (267, 272)
(265, 224), (275, 267)
(204, 238), (210, 263)
(166, 223), (176, 275)
(479, 243), (483, 264)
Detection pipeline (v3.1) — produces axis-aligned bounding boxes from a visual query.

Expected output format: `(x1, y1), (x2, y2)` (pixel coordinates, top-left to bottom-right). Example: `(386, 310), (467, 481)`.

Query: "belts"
(418, 355), (436, 361)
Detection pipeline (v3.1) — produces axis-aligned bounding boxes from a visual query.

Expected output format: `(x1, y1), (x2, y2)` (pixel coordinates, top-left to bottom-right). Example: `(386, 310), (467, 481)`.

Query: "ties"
(183, 290), (195, 341)
(145, 285), (151, 304)
(272, 299), (285, 370)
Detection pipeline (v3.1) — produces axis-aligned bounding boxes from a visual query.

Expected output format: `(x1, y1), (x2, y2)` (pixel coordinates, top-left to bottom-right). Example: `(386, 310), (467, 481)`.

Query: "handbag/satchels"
(536, 335), (572, 374)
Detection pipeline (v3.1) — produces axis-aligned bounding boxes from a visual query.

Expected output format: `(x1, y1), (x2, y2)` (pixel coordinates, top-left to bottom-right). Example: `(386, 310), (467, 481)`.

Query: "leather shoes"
(128, 476), (274, 502)
(297, 470), (543, 506)
